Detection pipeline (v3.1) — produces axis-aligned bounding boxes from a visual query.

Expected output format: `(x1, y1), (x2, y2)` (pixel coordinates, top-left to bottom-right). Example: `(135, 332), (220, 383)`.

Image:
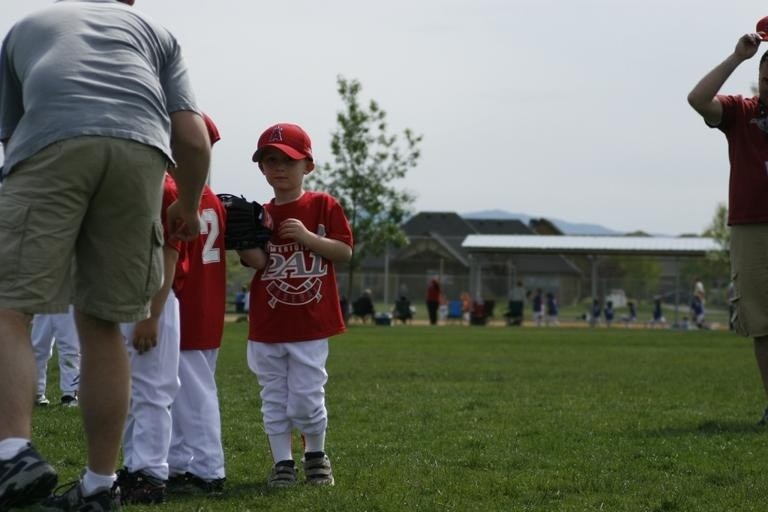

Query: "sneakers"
(34, 392), (52, 408)
(304, 451), (335, 488)
(270, 460), (299, 490)
(38, 478), (121, 512)
(166, 471), (226, 501)
(61, 392), (80, 408)
(0, 442), (59, 512)
(114, 465), (166, 505)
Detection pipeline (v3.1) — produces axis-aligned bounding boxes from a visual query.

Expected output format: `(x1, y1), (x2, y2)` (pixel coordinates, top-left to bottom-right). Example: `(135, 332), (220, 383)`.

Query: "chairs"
(339, 295), (525, 326)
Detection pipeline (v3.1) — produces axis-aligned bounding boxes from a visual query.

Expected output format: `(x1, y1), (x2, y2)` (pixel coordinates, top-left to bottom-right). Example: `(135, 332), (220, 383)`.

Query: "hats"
(252, 124), (314, 161)
(756, 15), (768, 41)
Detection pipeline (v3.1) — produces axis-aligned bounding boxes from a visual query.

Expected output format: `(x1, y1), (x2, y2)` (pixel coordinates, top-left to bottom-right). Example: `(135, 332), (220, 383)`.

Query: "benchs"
(539, 317), (666, 329)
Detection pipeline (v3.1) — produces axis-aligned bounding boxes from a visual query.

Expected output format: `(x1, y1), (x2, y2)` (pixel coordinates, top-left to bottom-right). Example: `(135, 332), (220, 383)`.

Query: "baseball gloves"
(219, 195), (276, 249)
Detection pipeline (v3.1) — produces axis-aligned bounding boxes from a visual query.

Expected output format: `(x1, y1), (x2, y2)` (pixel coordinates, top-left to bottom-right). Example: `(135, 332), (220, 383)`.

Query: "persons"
(393, 295), (412, 326)
(242, 290), (250, 323)
(163, 110), (228, 497)
(426, 279), (441, 325)
(120, 172), (182, 505)
(727, 281), (736, 330)
(687, 16), (767, 425)
(526, 281), (708, 330)
(506, 281), (527, 325)
(0, 0), (212, 509)
(235, 123), (353, 493)
(353, 289), (375, 325)
(234, 285), (246, 323)
(30, 301), (81, 409)
